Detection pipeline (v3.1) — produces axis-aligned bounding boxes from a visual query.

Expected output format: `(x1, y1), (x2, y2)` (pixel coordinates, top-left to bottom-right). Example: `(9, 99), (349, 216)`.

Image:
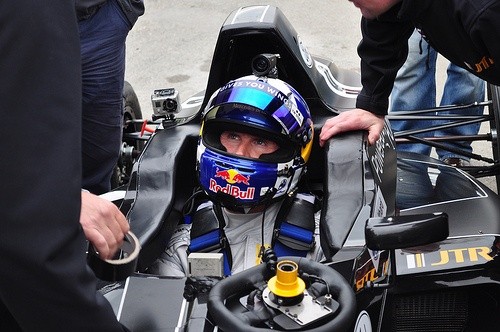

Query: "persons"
(0, 0), (145, 332)
(348, 0), (500, 178)
(80, 75), (386, 280)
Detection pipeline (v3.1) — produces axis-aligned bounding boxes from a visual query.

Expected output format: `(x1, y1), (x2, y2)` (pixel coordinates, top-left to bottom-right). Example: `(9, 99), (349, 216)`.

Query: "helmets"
(196, 75), (314, 208)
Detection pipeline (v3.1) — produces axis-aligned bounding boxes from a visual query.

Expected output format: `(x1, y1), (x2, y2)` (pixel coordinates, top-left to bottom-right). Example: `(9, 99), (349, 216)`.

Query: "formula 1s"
(84, 3), (499, 332)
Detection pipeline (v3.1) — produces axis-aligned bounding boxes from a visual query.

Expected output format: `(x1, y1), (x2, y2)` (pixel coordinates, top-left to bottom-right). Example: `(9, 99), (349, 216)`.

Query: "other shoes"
(436, 157), (476, 207)
(396, 153), (435, 209)
